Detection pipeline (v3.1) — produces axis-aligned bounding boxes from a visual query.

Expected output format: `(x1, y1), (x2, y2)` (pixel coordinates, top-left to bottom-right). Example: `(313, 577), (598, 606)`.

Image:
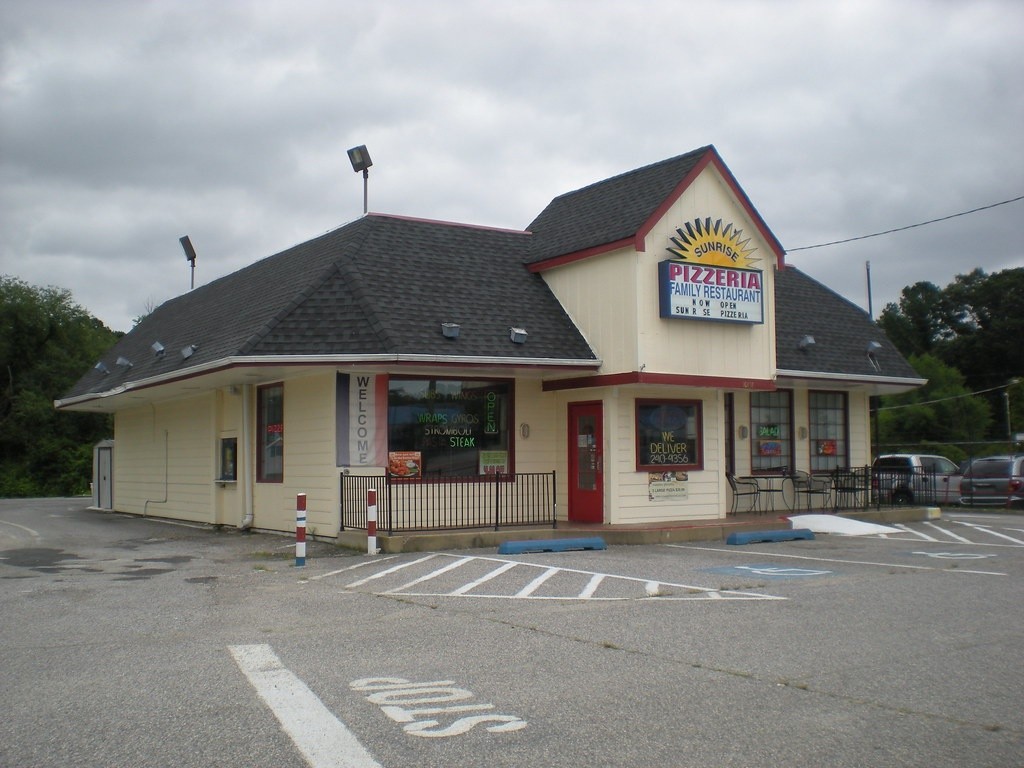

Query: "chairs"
(726, 472), (762, 515)
(791, 469), (874, 512)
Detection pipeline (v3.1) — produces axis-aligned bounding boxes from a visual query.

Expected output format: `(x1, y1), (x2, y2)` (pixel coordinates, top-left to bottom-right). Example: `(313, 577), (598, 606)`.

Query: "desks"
(813, 474), (866, 509)
(740, 475), (792, 513)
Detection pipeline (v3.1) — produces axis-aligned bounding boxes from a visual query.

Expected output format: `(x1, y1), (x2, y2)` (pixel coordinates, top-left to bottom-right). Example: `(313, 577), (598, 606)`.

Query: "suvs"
(959, 454), (1024, 511)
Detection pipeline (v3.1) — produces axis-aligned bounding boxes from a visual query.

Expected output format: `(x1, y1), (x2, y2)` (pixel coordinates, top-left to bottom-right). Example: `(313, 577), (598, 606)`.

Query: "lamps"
(347, 145), (373, 172)
(180, 236), (197, 261)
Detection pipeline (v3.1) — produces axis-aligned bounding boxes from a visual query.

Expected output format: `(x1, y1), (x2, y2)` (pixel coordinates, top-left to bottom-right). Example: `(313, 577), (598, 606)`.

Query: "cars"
(872, 454), (964, 507)
